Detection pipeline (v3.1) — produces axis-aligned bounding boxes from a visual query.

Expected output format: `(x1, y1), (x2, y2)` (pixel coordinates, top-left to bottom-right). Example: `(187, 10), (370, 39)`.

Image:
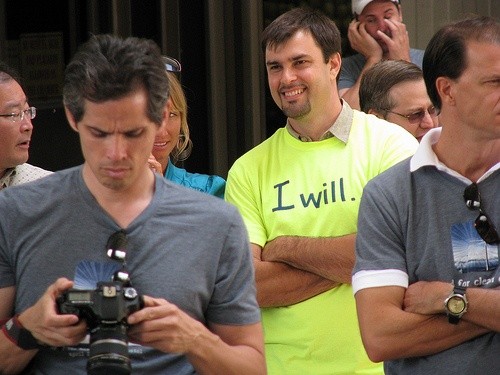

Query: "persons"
(351, 13), (500, 375)
(147, 71), (226, 201)
(0, 33), (267, 375)
(225, 8), (423, 375)
(335, 0), (426, 111)
(0, 71), (55, 192)
(359, 58), (440, 146)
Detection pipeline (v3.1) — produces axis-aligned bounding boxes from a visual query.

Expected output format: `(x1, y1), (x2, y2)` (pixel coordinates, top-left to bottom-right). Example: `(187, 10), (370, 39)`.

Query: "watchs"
(443, 285), (467, 325)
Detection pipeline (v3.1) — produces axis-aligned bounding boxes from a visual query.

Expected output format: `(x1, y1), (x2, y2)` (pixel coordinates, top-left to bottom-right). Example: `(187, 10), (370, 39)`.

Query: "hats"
(352, 0), (399, 16)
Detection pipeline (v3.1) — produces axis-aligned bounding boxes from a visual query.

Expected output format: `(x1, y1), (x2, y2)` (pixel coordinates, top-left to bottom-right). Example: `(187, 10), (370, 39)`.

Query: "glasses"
(160, 55), (181, 81)
(0, 106), (37, 123)
(381, 105), (437, 124)
(462, 181), (500, 248)
(105, 228), (132, 288)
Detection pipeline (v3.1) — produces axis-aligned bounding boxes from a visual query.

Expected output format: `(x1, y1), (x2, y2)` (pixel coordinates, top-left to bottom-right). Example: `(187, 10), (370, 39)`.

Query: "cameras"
(57, 281), (144, 375)
